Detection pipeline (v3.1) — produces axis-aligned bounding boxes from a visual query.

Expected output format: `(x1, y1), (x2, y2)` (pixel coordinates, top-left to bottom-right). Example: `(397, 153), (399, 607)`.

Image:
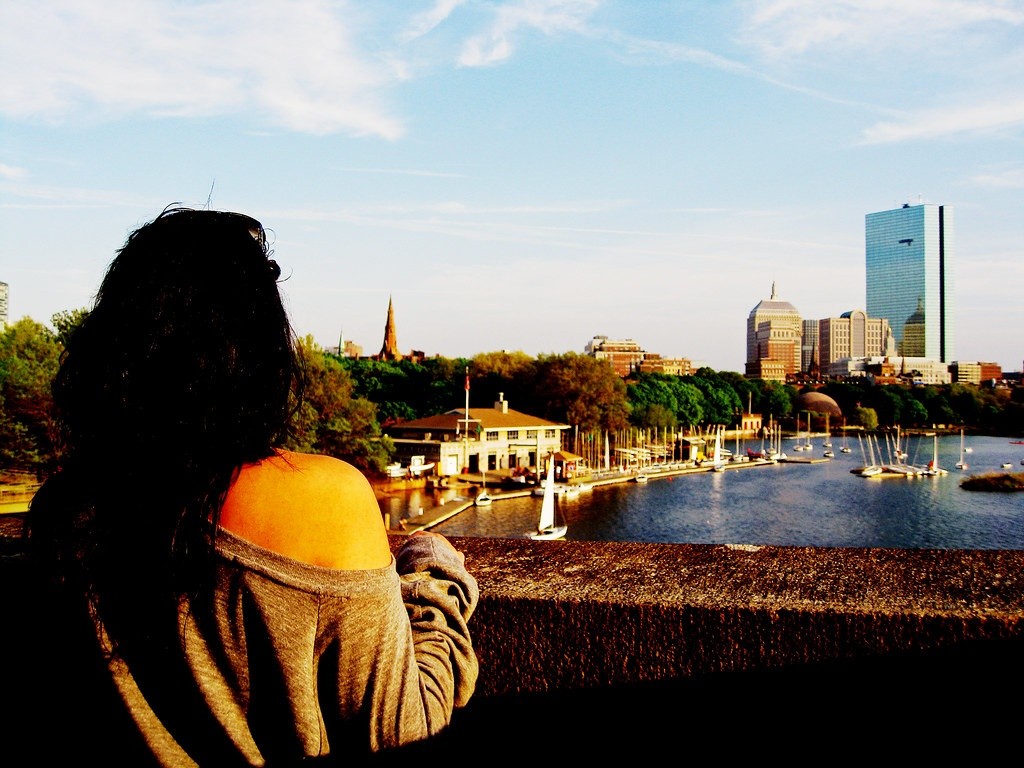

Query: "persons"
(0, 201), (481, 768)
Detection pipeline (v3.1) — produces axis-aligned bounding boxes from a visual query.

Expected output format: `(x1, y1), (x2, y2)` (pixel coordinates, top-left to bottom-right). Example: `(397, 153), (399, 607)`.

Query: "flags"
(464, 374), (470, 391)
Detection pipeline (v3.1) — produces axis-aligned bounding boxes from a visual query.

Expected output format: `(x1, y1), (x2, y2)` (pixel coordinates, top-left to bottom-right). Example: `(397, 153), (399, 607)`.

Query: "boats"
(1000, 462), (1014, 469)
(1009, 440), (1024, 444)
(474, 490), (492, 505)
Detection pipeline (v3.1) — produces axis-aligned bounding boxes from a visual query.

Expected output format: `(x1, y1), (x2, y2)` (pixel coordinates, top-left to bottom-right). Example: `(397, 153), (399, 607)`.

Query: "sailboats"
(535, 411), (949, 499)
(522, 453), (568, 540)
(954, 429), (969, 470)
